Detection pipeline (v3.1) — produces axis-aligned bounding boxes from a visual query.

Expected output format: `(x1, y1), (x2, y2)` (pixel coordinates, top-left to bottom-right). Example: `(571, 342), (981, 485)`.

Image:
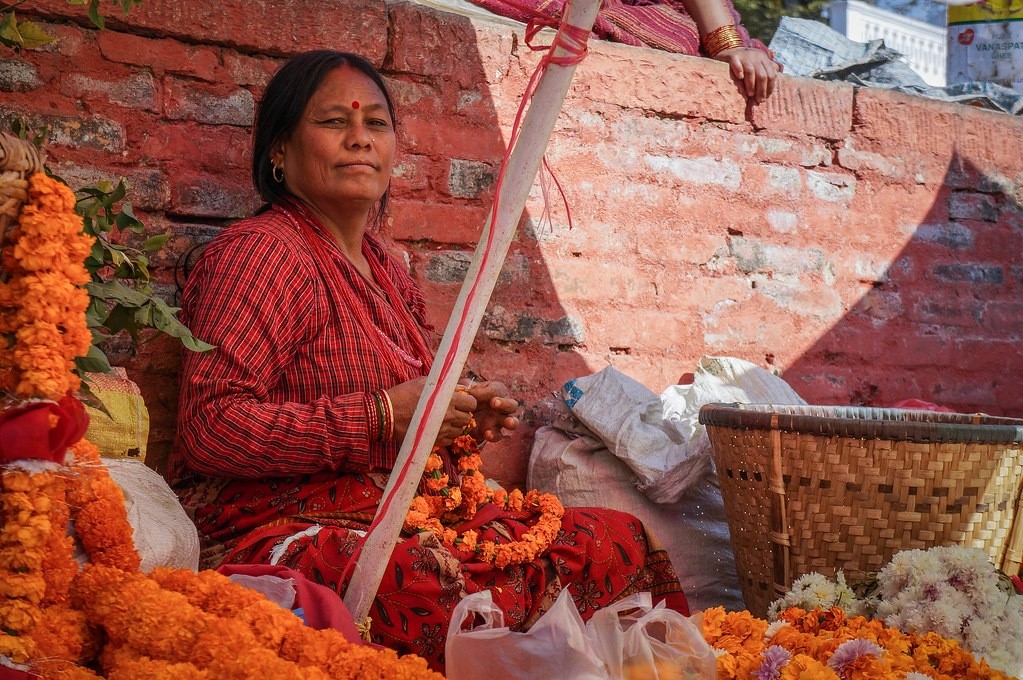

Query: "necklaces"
(274, 204), (423, 368)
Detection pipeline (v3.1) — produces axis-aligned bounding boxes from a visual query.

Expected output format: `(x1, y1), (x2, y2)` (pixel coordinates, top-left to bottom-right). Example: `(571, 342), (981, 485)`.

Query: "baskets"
(699, 402), (1023, 624)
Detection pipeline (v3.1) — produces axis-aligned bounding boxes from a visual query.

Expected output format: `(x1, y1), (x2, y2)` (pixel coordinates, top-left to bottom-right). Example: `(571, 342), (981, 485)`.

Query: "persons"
(167, 50), (692, 680)
(472, 0), (780, 103)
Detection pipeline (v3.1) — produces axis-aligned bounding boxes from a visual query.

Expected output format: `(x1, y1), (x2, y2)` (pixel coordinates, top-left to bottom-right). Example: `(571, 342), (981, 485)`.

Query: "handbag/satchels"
(586, 592), (718, 680)
(444, 584), (610, 680)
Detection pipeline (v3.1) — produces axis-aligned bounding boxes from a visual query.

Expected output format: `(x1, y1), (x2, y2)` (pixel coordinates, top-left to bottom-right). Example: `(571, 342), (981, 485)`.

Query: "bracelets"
(703, 26), (744, 57)
(365, 389), (394, 443)
